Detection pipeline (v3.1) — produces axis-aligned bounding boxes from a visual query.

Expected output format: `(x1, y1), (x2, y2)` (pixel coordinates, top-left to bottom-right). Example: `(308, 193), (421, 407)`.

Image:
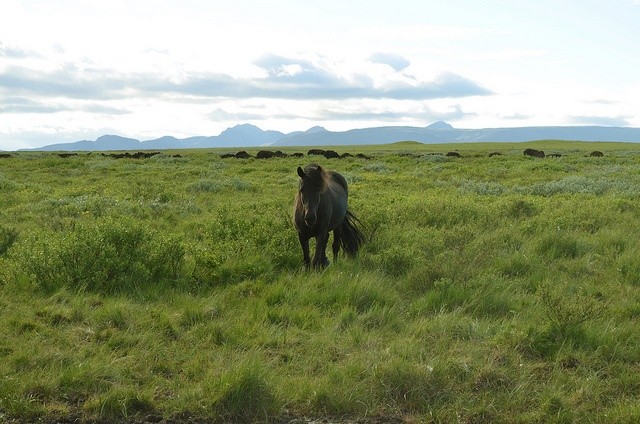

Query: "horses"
(292, 163), (372, 272)
